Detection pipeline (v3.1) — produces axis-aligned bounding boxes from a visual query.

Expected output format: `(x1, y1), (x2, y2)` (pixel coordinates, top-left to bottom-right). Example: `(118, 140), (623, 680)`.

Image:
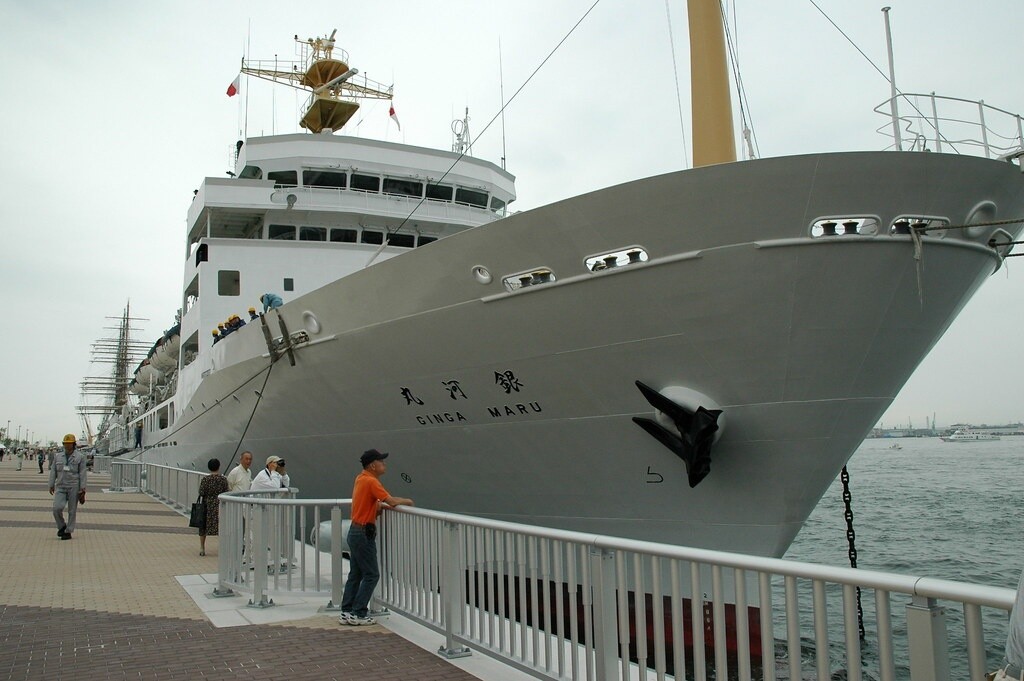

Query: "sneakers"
(339, 611), (351, 624)
(347, 612), (376, 625)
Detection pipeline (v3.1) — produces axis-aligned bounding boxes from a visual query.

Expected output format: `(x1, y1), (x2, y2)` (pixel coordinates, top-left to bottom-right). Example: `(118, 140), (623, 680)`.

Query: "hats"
(266, 456), (283, 465)
(360, 449), (388, 468)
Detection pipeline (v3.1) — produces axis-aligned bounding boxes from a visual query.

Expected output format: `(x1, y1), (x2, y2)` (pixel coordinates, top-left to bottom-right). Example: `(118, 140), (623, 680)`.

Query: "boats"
(938, 427), (1001, 442)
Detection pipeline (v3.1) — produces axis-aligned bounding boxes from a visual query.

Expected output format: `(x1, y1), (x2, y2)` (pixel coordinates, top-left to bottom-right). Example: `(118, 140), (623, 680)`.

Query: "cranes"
(909, 415), (912, 428)
(926, 416), (930, 430)
(931, 411), (936, 431)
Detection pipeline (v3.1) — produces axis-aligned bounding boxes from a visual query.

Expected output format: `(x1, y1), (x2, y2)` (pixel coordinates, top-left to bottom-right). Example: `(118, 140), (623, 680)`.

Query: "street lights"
(7, 420), (11, 440)
(26, 429), (29, 442)
(18, 425), (21, 442)
(32, 432), (35, 445)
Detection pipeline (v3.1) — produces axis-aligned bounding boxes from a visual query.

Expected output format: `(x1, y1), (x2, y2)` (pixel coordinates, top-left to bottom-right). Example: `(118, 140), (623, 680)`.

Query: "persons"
(198, 458), (228, 556)
(227, 452), (253, 554)
(339, 449), (414, 626)
(244, 455), (289, 570)
(48, 434), (87, 540)
(249, 307), (259, 321)
(260, 294), (284, 313)
(134, 424), (142, 448)
(0, 444), (54, 473)
(212, 314), (246, 344)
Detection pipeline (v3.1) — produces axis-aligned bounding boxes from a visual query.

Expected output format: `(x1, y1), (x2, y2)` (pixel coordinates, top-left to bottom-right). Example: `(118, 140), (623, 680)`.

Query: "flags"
(390, 105), (400, 129)
(226, 74), (240, 97)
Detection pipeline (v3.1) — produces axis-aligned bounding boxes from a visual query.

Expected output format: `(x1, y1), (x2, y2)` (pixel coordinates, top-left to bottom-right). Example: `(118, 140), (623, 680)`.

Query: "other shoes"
(199, 552), (205, 556)
(268, 567), (286, 573)
(16, 469), (21, 471)
(61, 533), (71, 540)
(57, 523), (66, 536)
(282, 562), (297, 568)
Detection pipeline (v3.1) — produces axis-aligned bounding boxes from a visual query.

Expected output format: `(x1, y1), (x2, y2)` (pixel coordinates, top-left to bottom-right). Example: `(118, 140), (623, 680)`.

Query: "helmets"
(62, 434), (75, 443)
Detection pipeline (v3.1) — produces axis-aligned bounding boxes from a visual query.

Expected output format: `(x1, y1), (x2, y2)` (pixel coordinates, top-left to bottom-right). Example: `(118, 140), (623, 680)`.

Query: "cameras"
(277, 460), (286, 467)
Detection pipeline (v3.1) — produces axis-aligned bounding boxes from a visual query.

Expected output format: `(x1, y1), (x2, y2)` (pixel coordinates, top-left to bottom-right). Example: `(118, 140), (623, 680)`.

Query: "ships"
(72, 2), (1024, 672)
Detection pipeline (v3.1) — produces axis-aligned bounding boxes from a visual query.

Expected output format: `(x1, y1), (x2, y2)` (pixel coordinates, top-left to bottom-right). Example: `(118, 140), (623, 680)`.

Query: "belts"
(351, 522), (366, 528)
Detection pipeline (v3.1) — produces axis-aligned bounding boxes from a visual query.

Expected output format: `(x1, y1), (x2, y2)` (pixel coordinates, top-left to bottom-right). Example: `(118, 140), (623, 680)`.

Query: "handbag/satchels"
(189, 495), (203, 527)
